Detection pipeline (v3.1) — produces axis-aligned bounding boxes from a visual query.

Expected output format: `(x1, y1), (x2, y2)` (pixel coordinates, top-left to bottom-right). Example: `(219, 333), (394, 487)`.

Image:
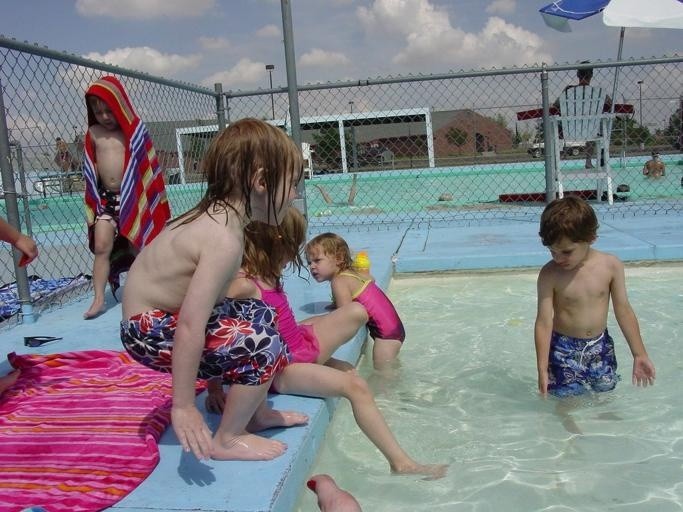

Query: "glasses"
(24, 337), (62, 346)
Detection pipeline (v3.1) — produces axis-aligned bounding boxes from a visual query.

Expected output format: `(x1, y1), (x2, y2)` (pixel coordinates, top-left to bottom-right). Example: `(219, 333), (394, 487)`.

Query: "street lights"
(267, 65), (276, 120)
(638, 80), (643, 127)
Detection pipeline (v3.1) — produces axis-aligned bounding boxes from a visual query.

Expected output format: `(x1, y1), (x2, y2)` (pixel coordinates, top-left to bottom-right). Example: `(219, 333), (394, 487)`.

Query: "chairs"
(531, 85), (616, 205)
(381, 150), (394, 170)
(301, 142), (315, 180)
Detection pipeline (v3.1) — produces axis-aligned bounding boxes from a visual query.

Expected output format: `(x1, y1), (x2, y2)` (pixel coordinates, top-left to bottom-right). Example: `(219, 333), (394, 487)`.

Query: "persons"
(204, 204), (450, 482)
(552, 60), (613, 168)
(616, 184), (634, 202)
(533, 195), (656, 439)
(439, 193), (452, 201)
(82, 75), (171, 319)
(119, 117), (311, 462)
(643, 151), (665, 183)
(311, 173), (359, 208)
(303, 232), (406, 369)
(0, 368), (23, 393)
(306, 474), (360, 512)
(0, 216), (39, 268)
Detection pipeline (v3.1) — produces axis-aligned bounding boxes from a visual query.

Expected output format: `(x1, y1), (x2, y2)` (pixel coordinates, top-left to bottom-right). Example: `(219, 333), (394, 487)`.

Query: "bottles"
(353, 252), (369, 277)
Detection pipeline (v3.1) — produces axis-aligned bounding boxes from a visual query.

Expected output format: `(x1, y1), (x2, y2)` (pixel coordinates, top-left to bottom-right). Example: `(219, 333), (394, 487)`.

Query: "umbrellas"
(539, 1), (682, 114)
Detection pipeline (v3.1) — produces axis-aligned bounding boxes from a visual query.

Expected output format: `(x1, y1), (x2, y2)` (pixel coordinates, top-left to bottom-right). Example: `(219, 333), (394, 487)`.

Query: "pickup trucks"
(528, 140), (585, 160)
